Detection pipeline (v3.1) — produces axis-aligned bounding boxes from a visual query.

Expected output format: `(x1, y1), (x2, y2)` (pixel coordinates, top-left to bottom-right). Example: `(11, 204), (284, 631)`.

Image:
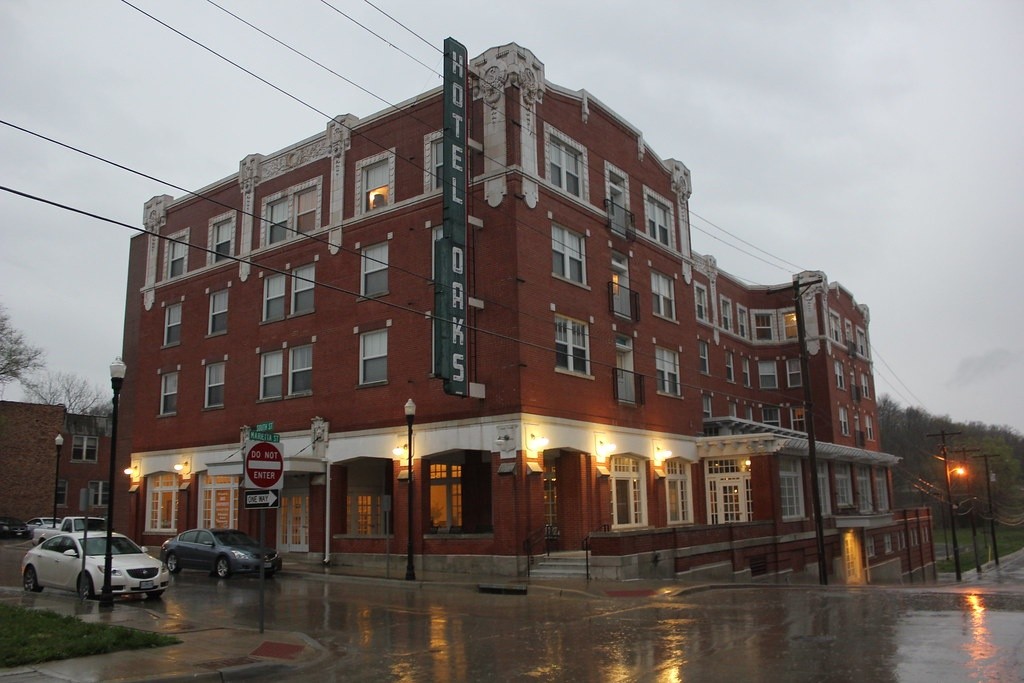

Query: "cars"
(0, 517), (28, 539)
(21, 531), (170, 600)
(159, 528), (283, 579)
(25, 517), (63, 538)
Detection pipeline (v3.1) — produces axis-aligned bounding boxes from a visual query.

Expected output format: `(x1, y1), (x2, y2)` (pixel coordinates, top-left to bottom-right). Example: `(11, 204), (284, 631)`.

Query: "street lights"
(99, 356), (128, 607)
(403, 398), (416, 582)
(53, 432), (64, 528)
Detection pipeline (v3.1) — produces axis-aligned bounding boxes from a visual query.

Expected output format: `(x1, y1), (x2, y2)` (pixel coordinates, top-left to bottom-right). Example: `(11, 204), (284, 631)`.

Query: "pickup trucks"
(32, 517), (107, 546)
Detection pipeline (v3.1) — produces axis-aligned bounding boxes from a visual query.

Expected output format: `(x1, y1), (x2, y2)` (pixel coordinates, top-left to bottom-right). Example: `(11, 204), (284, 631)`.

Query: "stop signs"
(246, 442), (284, 489)
